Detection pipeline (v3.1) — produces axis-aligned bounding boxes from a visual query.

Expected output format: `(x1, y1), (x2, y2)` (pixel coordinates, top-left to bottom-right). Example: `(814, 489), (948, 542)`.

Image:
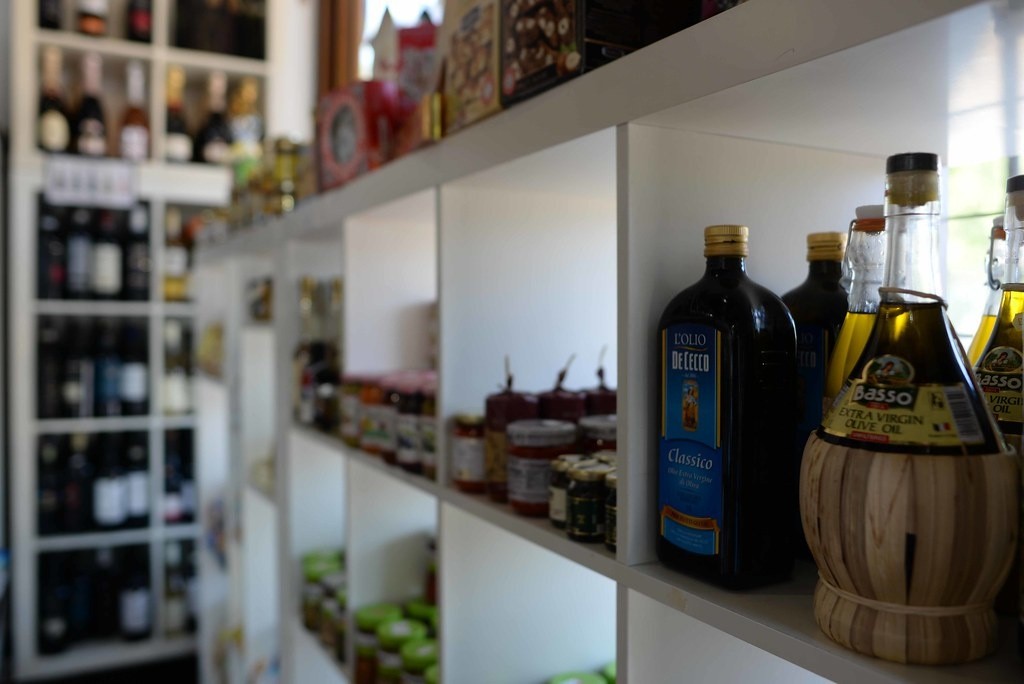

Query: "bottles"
(40, 318), (189, 416)
(780, 235), (850, 565)
(41, 542), (198, 653)
(39, 0), (265, 166)
(966, 216), (1005, 370)
(37, 196), (247, 301)
(654, 224), (800, 590)
(798, 152), (1017, 666)
(822, 204), (887, 426)
(972, 175), (1024, 619)
(39, 431), (195, 531)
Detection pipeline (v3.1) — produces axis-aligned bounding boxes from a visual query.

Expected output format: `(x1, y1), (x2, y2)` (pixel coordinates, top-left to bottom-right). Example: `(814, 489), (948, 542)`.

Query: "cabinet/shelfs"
(6, 0), (318, 682)
(193, 0), (1024, 684)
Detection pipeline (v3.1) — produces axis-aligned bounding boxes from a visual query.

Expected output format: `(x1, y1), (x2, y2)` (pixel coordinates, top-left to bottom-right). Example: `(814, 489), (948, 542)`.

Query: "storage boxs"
(318, 0), (745, 191)
